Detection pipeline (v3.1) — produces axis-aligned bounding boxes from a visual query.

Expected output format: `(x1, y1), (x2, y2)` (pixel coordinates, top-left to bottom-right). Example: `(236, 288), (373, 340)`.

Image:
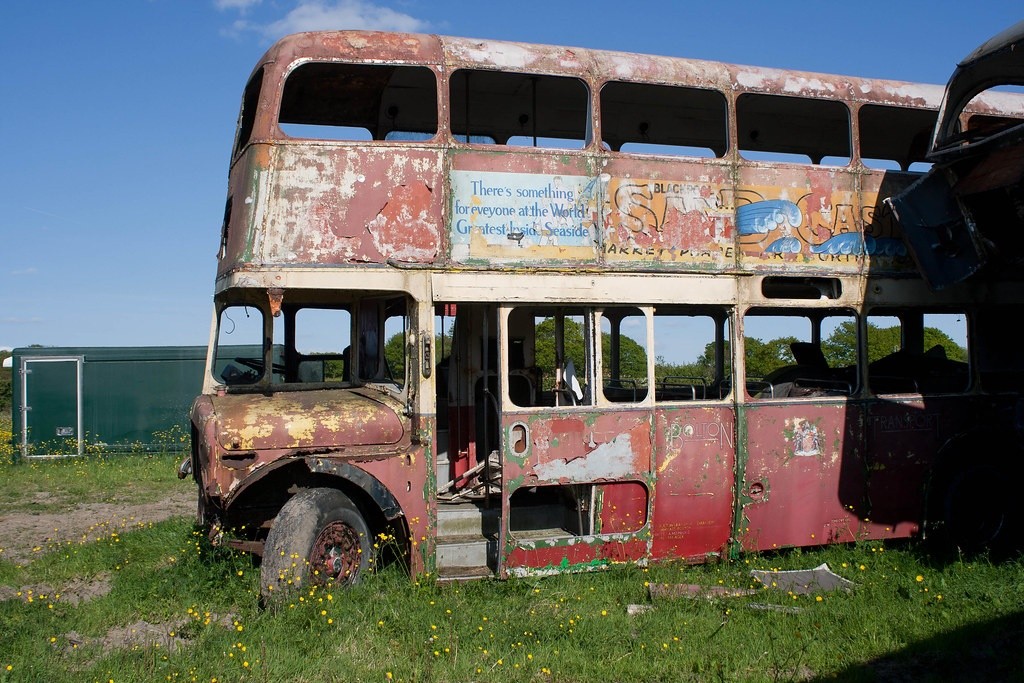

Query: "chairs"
(341, 344), (357, 381)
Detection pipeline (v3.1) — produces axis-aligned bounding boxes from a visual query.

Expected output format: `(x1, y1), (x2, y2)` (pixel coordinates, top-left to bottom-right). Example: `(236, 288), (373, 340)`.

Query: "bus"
(178, 30), (1023, 611)
